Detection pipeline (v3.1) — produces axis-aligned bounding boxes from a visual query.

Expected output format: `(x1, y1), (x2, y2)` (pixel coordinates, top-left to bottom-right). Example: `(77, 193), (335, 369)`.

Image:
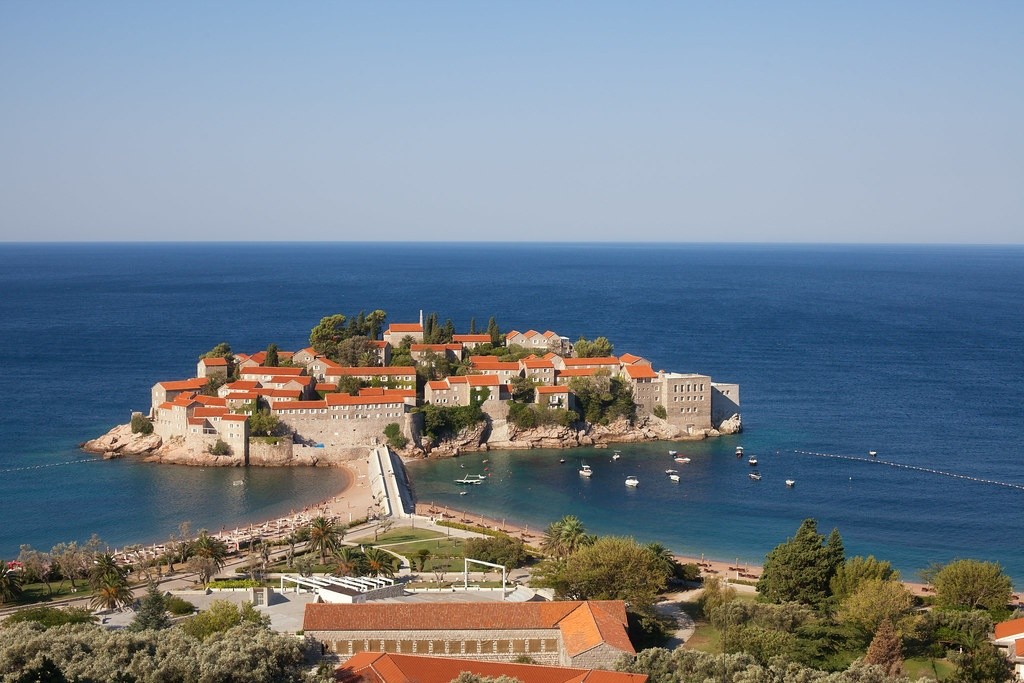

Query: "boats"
(625, 475), (639, 487)
(612, 450), (622, 461)
(454, 473), (487, 485)
(664, 446), (795, 486)
(579, 464), (594, 477)
